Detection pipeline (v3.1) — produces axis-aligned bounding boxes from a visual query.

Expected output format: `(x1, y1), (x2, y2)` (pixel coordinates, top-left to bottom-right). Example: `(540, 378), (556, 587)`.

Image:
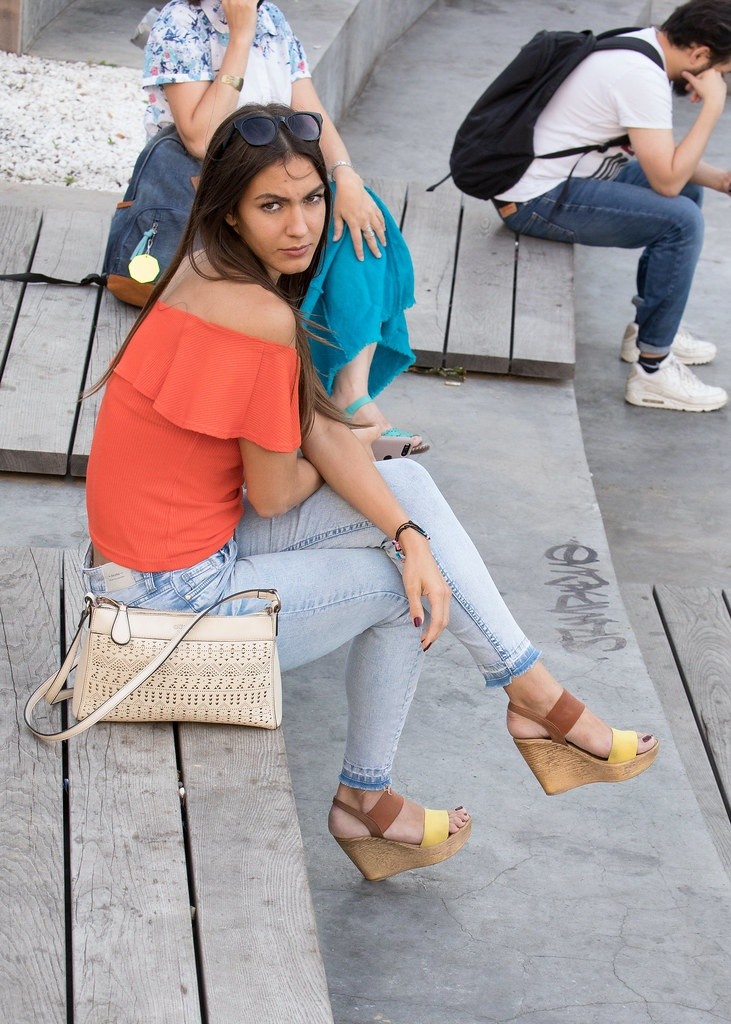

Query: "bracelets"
(393, 521), (430, 563)
(329, 161), (352, 183)
(221, 74), (244, 91)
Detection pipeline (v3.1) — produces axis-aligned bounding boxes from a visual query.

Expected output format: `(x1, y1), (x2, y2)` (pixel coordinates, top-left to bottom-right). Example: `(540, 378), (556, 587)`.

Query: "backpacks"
(107, 122), (210, 308)
(451, 27), (663, 200)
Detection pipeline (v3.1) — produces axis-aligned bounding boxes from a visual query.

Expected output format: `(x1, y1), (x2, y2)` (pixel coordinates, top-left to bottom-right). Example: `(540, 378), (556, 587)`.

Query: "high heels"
(506, 691), (659, 797)
(331, 788), (472, 880)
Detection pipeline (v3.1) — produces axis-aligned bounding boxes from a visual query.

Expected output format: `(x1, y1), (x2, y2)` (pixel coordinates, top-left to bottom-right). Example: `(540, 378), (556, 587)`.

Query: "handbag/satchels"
(22, 588), (283, 742)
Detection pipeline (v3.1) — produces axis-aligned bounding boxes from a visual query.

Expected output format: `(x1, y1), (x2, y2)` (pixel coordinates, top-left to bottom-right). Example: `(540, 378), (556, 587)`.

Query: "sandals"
(341, 393), (428, 455)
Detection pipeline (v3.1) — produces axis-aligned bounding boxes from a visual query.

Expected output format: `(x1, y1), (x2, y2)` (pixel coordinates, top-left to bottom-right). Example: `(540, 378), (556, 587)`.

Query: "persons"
(79, 103), (660, 882)
(144, 0), (429, 455)
(491, 0), (731, 412)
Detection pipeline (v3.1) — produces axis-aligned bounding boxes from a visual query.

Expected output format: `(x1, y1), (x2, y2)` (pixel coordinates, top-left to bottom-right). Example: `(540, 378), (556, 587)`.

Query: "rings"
(364, 225), (374, 236)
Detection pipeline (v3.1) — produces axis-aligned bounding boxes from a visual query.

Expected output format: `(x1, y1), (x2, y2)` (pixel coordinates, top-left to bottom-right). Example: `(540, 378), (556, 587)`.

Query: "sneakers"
(619, 320), (717, 366)
(625, 353), (728, 412)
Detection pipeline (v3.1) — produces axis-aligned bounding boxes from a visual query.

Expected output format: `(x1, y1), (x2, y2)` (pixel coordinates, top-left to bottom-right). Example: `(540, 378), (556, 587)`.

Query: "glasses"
(221, 111), (324, 154)
